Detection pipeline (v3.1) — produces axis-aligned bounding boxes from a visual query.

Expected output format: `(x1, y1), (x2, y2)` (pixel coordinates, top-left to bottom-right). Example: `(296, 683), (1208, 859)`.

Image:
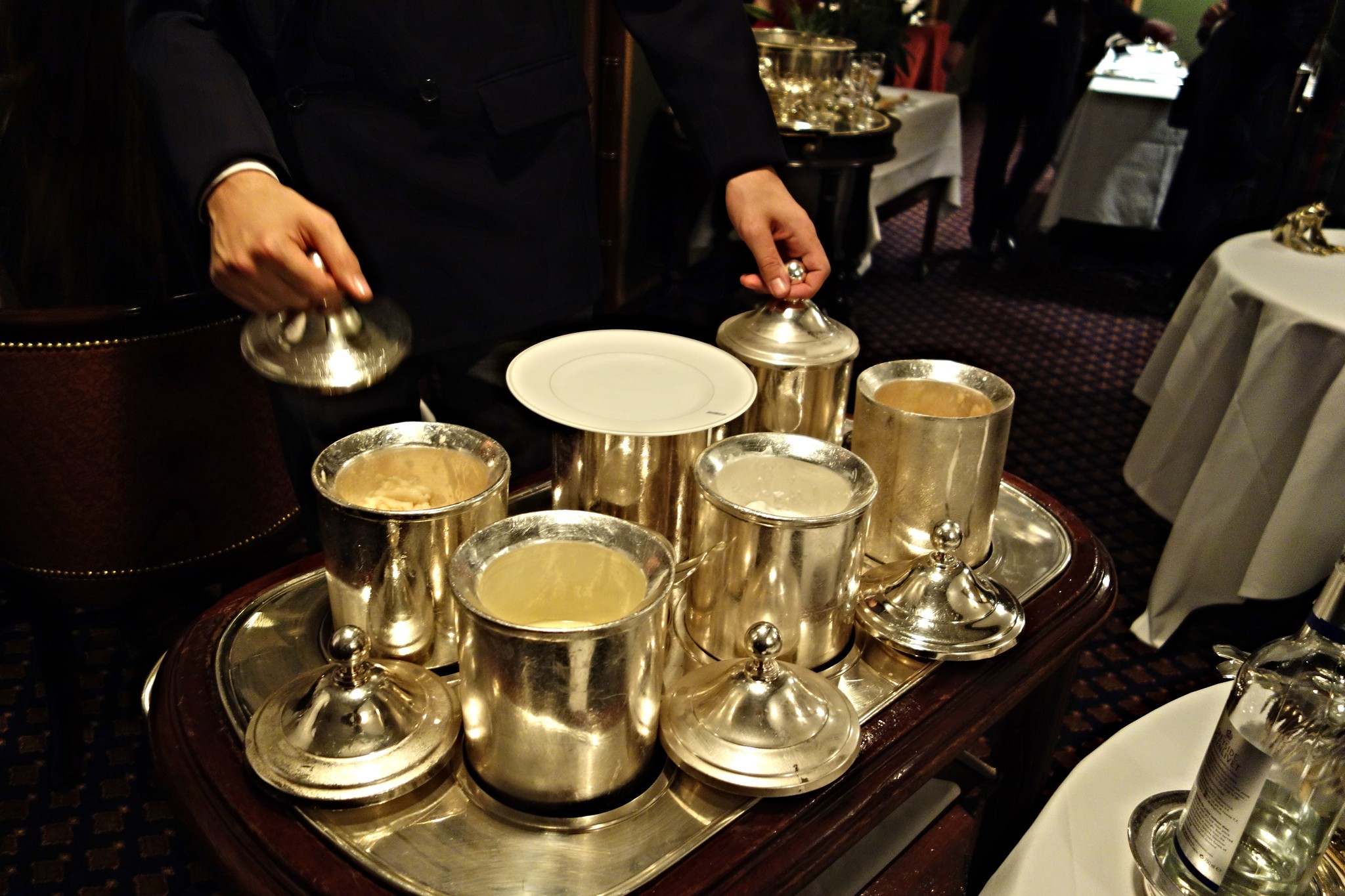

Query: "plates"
(507, 329), (760, 436)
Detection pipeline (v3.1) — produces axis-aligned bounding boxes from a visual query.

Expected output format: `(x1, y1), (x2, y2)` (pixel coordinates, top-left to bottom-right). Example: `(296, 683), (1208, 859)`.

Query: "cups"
(311, 422), (511, 669)
(684, 432), (877, 668)
(851, 358), (1016, 569)
(548, 419), (727, 566)
(448, 509), (723, 804)
(730, 363), (853, 450)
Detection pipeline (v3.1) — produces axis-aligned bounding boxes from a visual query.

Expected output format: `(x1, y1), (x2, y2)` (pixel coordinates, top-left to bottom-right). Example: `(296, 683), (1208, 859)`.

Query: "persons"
(126, 0), (832, 554)
(941, 1), (1175, 270)
(1130, 1), (1337, 312)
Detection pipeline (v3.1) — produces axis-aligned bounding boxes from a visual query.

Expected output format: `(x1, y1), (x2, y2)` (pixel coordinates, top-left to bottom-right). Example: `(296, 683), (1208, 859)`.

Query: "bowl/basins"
(1128, 790), (1345, 896)
(753, 26), (857, 79)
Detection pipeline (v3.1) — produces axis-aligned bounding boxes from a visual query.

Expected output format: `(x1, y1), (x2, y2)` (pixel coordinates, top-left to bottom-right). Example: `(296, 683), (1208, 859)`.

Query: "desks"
(146, 18), (1344, 896)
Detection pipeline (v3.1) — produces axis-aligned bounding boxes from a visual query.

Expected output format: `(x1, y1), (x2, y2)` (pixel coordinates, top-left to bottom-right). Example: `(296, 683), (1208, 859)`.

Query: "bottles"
(1165, 544), (1345, 895)
